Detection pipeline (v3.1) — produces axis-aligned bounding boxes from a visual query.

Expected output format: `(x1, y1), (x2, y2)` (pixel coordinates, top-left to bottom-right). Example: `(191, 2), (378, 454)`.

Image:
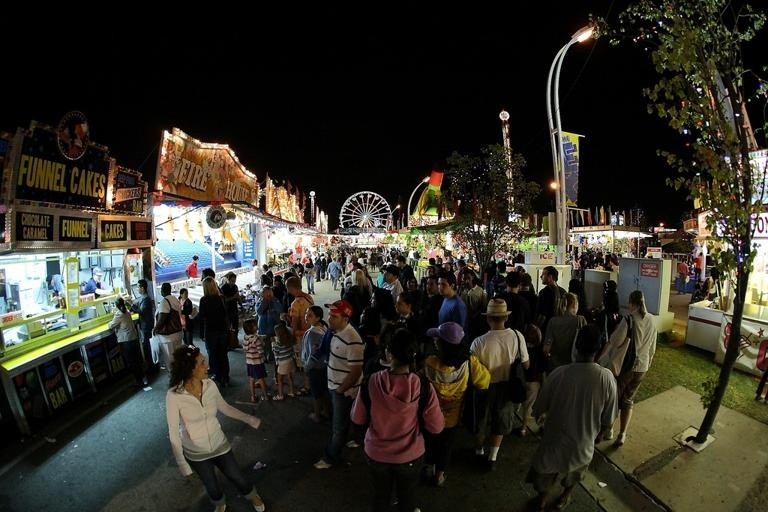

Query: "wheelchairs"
(690, 280), (707, 305)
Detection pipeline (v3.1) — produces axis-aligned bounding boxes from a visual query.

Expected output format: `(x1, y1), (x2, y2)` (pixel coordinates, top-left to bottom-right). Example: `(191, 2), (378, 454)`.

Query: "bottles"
(80, 290), (97, 304)
(0, 296), (19, 318)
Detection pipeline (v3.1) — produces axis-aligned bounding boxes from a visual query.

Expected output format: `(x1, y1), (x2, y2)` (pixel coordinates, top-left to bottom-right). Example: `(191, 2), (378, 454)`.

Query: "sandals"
(295, 386), (312, 396)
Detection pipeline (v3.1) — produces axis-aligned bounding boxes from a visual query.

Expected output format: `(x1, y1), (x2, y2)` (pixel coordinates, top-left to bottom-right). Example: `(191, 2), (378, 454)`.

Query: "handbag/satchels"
(508, 355), (529, 404)
(152, 308), (182, 335)
(187, 307), (198, 320)
(596, 314), (636, 377)
(148, 329), (161, 364)
(225, 327), (240, 348)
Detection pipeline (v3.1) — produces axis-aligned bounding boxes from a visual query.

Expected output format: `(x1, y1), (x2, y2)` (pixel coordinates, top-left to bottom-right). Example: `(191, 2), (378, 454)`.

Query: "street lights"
(546, 23), (599, 265)
(389, 205), (400, 232)
(407, 176), (430, 228)
(309, 190), (316, 227)
(499, 110), (515, 244)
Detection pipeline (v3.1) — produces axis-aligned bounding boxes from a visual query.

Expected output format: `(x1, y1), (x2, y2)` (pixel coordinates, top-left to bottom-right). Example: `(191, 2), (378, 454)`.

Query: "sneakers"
(612, 433), (626, 448)
(412, 425), (572, 512)
(344, 440), (362, 448)
(312, 459), (335, 469)
(248, 377), (295, 402)
(605, 429), (614, 440)
(309, 409), (331, 422)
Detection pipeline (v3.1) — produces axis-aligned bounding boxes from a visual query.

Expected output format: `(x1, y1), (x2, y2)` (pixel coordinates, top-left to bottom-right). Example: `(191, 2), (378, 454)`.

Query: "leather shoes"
(214, 503), (227, 512)
(252, 494), (266, 512)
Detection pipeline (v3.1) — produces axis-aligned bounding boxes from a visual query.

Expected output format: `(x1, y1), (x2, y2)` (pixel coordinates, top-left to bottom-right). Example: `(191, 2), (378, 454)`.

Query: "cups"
(114, 284), (119, 294)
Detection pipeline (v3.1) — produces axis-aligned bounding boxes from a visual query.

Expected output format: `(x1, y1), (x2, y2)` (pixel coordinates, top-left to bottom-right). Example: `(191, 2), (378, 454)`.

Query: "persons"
(755, 367), (768, 404)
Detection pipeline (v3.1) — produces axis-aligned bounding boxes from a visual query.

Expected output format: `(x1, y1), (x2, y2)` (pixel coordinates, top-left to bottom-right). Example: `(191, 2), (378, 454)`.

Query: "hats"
(426, 321), (466, 346)
(323, 300), (354, 318)
(480, 297), (513, 317)
